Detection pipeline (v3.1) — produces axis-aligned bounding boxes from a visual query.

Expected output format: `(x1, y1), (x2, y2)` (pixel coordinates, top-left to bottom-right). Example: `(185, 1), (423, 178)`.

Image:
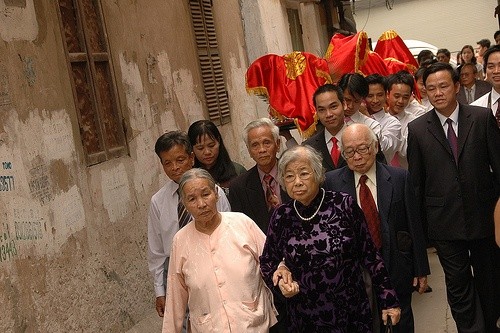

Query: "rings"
(421, 277), (425, 280)
(289, 272), (292, 276)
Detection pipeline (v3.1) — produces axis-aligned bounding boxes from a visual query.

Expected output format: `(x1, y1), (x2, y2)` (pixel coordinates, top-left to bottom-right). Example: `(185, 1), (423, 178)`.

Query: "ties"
(330, 137), (340, 166)
(264, 173), (280, 212)
(497, 98), (500, 123)
(445, 118), (457, 163)
(358, 174), (381, 250)
(177, 187), (194, 229)
(465, 88), (473, 104)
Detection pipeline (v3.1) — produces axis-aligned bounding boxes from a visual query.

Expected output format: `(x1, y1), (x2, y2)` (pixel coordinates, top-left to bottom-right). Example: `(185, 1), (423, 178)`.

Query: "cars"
(371, 40), (458, 68)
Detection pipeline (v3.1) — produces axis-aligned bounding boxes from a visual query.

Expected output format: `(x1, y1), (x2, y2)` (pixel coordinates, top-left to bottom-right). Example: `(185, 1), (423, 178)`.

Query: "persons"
(325, 124), (431, 333)
(187, 120), (247, 197)
(280, 30), (500, 294)
(229, 118), (293, 237)
(147, 130), (231, 333)
(494, 0), (500, 29)
(405, 62), (500, 333)
(162, 169), (293, 333)
(259, 145), (402, 333)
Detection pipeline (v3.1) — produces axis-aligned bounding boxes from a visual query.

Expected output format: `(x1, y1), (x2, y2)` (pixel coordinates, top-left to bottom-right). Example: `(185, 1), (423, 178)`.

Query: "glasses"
(281, 171), (315, 182)
(342, 140), (373, 158)
(461, 72), (472, 77)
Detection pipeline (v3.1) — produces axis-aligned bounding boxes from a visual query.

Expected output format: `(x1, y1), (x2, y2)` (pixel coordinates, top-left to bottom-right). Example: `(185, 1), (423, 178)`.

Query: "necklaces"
(294, 188), (325, 220)
